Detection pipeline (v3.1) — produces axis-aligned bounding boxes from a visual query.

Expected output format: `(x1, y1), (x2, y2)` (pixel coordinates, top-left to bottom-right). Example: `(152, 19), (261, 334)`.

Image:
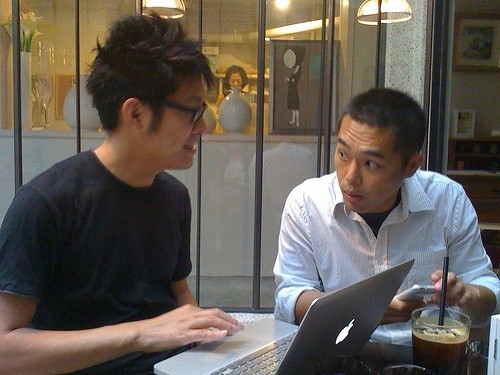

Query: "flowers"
(5, 11), (42, 53)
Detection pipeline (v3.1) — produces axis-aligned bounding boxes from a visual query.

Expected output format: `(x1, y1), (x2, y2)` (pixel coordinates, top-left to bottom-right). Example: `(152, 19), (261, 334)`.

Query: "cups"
(312, 355), (371, 375)
(410, 306), (472, 375)
(465, 338), (489, 375)
(35, 40), (56, 131)
(376, 364), (434, 375)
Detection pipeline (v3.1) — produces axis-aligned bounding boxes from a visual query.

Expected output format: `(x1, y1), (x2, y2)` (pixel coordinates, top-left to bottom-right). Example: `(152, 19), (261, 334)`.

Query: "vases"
(20, 52), (32, 131)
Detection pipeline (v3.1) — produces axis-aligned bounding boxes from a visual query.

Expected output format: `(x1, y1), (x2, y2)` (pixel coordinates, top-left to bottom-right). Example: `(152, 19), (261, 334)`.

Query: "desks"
(189, 326), (489, 375)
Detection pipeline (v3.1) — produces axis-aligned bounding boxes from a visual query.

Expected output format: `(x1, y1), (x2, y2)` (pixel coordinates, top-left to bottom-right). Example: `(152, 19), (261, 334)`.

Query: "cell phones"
(397, 284), (439, 302)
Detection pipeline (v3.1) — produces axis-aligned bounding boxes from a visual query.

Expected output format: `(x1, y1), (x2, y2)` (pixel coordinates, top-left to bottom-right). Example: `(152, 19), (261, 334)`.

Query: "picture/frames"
(451, 13), (500, 73)
(452, 108), (475, 139)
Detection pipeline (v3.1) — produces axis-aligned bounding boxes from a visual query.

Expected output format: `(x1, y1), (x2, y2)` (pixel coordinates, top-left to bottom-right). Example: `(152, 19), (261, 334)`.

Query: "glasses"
(162, 98), (206, 123)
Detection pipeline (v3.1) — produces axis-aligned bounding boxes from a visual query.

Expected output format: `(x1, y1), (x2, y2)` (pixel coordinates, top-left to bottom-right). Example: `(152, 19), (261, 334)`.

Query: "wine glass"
(31, 72), (52, 132)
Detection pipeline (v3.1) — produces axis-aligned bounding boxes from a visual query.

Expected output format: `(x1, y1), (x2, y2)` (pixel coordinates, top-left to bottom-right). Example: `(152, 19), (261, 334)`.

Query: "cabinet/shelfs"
(447, 137), (500, 268)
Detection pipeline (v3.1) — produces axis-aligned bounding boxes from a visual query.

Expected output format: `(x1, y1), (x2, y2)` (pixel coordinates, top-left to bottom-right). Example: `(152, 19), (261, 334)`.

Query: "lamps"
(142, 0), (186, 19)
(356, 0), (412, 25)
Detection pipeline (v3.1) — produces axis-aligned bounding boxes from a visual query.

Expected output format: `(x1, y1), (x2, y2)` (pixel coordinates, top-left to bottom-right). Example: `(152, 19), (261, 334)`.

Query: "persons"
(215, 65), (251, 107)
(0, 12), (246, 375)
(271, 89), (500, 348)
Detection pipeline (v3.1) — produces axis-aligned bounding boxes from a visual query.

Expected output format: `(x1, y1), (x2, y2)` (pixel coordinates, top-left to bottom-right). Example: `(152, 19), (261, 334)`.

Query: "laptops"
(152, 258), (416, 375)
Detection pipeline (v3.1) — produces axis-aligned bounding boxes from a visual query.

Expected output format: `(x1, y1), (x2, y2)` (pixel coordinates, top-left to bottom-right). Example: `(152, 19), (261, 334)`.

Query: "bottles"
(63, 76), (102, 132)
(203, 100), (217, 134)
(218, 86), (252, 135)
